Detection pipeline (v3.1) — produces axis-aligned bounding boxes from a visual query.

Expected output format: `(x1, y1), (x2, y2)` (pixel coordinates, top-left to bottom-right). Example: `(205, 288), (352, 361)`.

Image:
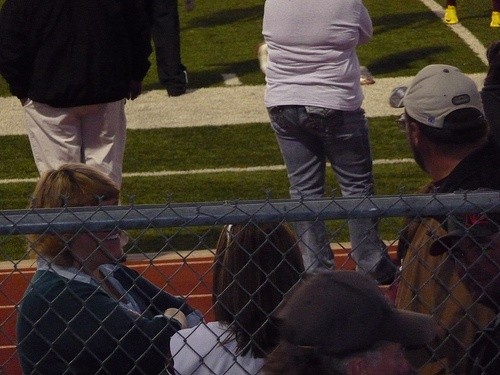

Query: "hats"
(429, 187), (500, 256)
(268, 268), (436, 352)
(397, 64), (487, 129)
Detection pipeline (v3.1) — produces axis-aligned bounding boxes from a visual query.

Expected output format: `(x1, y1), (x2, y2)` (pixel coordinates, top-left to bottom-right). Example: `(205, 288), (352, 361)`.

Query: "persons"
(15, 166), (204, 375)
(165, 218), (321, 375)
(257, 270), (438, 375)
(445, 0), (500, 28)
(0, 0), (186, 264)
(386, 64), (500, 375)
(260, 0), (399, 290)
(477, 44), (499, 196)
(427, 205), (499, 375)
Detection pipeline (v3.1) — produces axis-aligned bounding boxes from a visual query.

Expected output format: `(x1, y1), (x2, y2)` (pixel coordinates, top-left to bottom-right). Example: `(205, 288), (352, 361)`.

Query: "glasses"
(395, 114), (417, 131)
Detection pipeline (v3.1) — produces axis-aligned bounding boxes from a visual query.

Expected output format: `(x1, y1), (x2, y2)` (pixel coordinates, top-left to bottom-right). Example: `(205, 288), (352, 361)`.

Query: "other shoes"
(364, 256), (400, 288)
(168, 85), (185, 96)
(443, 5), (458, 24)
(490, 11), (500, 27)
